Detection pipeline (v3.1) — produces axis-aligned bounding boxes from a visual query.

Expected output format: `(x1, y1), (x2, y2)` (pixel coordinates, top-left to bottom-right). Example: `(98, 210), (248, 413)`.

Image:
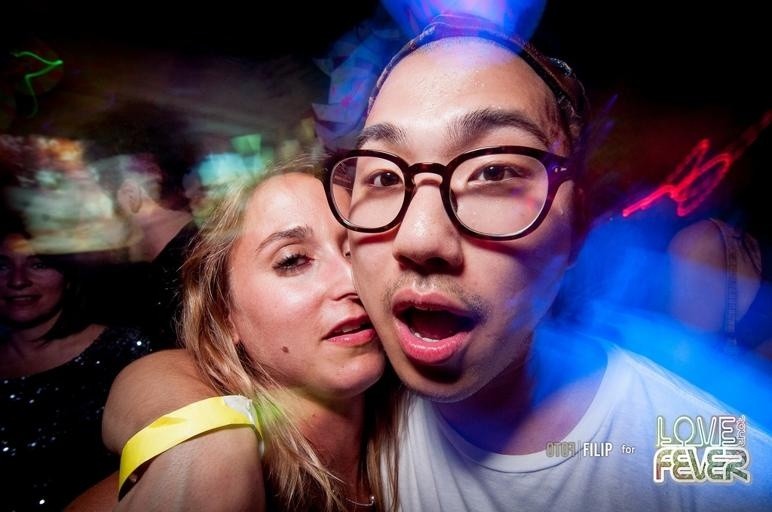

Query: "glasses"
(319, 146), (584, 243)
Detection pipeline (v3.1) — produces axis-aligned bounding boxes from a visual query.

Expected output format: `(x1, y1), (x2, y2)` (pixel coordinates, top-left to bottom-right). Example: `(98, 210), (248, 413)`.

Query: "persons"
(0, 12), (770, 511)
